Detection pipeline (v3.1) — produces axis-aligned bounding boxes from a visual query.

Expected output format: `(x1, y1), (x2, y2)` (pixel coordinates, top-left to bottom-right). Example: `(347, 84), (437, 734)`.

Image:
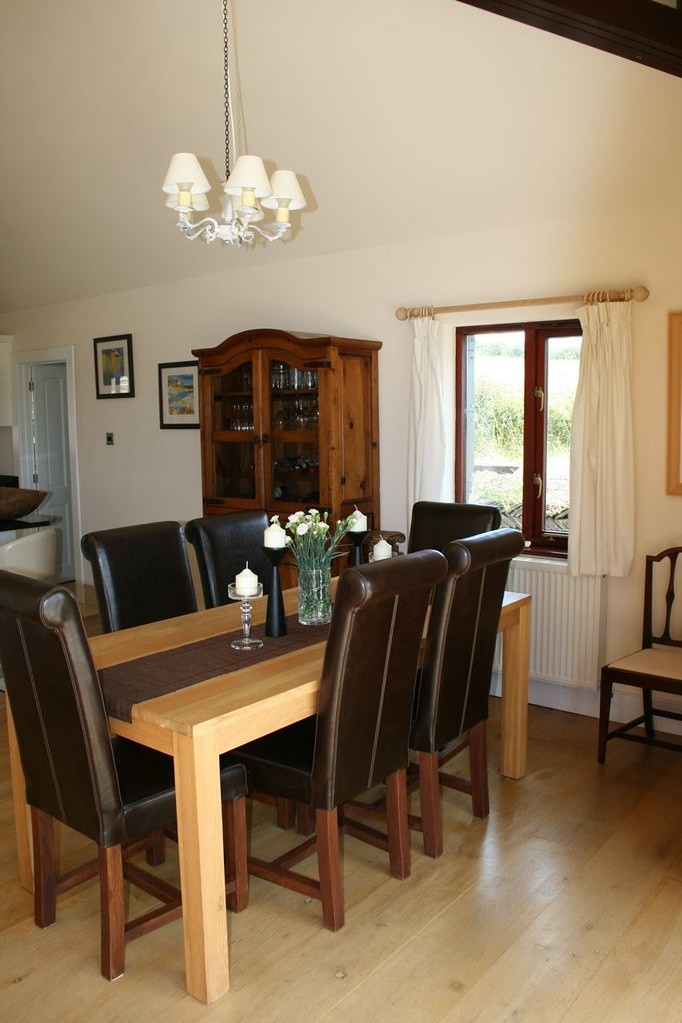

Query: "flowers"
(270, 507), (361, 565)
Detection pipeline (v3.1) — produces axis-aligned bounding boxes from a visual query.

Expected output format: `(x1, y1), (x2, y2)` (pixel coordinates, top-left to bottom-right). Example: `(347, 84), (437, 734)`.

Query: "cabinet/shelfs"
(191, 329), (384, 591)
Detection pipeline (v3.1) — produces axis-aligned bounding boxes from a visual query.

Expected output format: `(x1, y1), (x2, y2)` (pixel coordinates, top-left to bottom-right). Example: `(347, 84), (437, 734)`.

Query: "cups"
(240, 359), (318, 390)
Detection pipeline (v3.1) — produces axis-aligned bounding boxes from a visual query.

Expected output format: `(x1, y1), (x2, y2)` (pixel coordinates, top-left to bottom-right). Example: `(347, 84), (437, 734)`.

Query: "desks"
(3, 577), (533, 1002)
(0, 513), (58, 533)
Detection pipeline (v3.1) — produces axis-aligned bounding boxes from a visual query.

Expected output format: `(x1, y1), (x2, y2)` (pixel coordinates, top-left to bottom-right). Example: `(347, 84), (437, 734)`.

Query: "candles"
(373, 535), (395, 560)
(349, 505), (366, 531)
(234, 560), (257, 598)
(265, 516), (289, 548)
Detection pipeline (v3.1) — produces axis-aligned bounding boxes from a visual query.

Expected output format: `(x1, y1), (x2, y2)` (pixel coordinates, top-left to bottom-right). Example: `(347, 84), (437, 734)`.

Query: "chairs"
(0, 529), (60, 584)
(380, 500), (503, 785)
(597, 548), (682, 761)
(224, 549), (453, 928)
(185, 510), (297, 829)
(0, 570), (256, 980)
(349, 527), (526, 857)
(79, 523), (204, 858)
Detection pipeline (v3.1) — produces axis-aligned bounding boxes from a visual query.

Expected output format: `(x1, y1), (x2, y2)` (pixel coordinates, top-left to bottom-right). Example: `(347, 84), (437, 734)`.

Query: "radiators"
(490, 556), (609, 693)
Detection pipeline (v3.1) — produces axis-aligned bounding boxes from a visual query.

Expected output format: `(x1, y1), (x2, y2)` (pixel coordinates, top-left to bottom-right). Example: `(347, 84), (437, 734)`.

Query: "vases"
(297, 564), (332, 624)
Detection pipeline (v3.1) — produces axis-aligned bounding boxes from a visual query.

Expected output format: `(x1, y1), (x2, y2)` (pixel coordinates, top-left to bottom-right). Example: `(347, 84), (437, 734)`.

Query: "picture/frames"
(158, 360), (201, 430)
(93, 333), (135, 400)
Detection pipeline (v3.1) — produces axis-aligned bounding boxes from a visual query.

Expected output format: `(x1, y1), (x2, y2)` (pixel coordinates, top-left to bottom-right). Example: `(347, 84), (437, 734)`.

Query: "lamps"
(162, 0), (307, 245)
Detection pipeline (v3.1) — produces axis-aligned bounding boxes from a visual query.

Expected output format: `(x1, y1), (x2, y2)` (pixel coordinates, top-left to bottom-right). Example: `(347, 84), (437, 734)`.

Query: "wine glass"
(227, 393), (319, 432)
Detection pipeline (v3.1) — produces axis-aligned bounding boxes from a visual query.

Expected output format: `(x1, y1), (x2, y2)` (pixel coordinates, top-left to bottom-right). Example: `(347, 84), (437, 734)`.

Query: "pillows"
(0, 488), (49, 520)
